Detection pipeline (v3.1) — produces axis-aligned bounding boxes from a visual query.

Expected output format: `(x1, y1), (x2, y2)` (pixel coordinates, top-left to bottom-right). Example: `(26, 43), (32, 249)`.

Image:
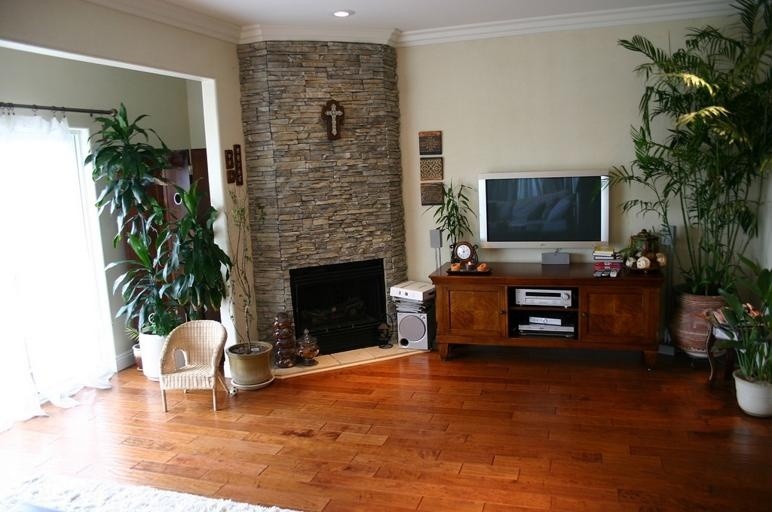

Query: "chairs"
(155, 319), (228, 410)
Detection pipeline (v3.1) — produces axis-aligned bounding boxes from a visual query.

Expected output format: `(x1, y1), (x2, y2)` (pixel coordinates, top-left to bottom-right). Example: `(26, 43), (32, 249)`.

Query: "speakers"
(396, 312), (435, 351)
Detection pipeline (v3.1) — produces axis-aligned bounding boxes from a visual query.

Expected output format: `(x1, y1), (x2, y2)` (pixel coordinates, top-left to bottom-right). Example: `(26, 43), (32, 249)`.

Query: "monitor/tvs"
(477, 170), (611, 250)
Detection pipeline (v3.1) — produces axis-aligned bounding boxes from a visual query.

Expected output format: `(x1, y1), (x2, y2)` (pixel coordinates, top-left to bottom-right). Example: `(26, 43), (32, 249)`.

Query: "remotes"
(593, 268), (620, 278)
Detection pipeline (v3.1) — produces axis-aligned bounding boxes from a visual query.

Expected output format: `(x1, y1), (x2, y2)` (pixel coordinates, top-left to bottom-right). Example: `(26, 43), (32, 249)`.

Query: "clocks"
(450, 240), (478, 268)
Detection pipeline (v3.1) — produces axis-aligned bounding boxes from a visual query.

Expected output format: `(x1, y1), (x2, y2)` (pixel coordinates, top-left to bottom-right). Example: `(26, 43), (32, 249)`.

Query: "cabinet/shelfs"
(427, 262), (673, 370)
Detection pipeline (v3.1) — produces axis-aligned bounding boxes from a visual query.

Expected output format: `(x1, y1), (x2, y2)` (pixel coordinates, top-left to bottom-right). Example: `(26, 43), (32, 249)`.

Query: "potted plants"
(83, 105), (184, 382)
(214, 182), (280, 390)
(694, 281), (770, 419)
(614, 2), (771, 359)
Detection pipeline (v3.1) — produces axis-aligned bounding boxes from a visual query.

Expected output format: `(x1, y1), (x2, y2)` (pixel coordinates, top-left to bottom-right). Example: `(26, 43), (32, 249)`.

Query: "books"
(393, 298), (426, 313)
(592, 246), (624, 263)
